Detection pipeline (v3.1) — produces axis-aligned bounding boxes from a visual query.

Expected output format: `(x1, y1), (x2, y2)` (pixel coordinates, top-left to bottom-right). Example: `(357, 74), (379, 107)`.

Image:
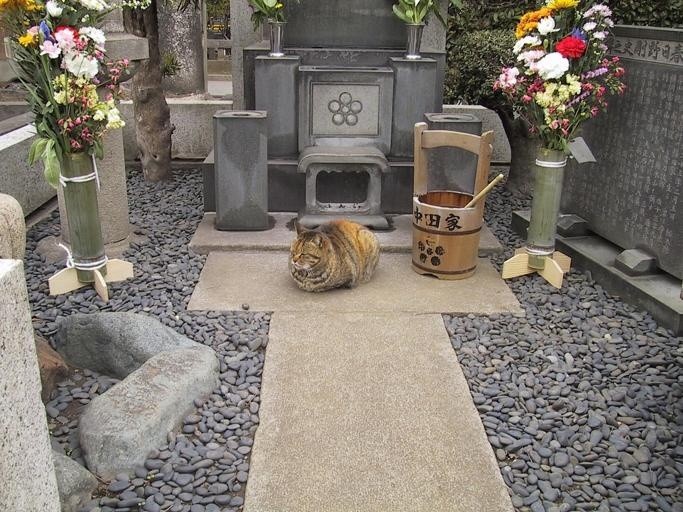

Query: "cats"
(288, 218), (381, 294)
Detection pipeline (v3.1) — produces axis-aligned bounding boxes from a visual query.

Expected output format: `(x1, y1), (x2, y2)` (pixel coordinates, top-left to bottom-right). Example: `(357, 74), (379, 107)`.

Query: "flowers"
(490, 1), (632, 159)
(0, 0), (157, 199)
(245, 0), (289, 35)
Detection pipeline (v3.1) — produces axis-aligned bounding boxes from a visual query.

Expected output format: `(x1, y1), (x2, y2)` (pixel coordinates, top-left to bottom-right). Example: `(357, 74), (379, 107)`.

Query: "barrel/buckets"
(412, 120), (494, 282)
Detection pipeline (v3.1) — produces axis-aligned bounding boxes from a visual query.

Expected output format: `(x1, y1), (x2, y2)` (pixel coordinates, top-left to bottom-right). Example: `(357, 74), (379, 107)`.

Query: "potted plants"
(391, 1), (465, 61)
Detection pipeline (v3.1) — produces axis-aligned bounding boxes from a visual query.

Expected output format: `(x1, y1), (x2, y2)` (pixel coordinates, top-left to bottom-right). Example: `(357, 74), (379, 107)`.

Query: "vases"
(44, 148), (134, 305)
(265, 20), (288, 58)
(499, 145), (572, 290)
